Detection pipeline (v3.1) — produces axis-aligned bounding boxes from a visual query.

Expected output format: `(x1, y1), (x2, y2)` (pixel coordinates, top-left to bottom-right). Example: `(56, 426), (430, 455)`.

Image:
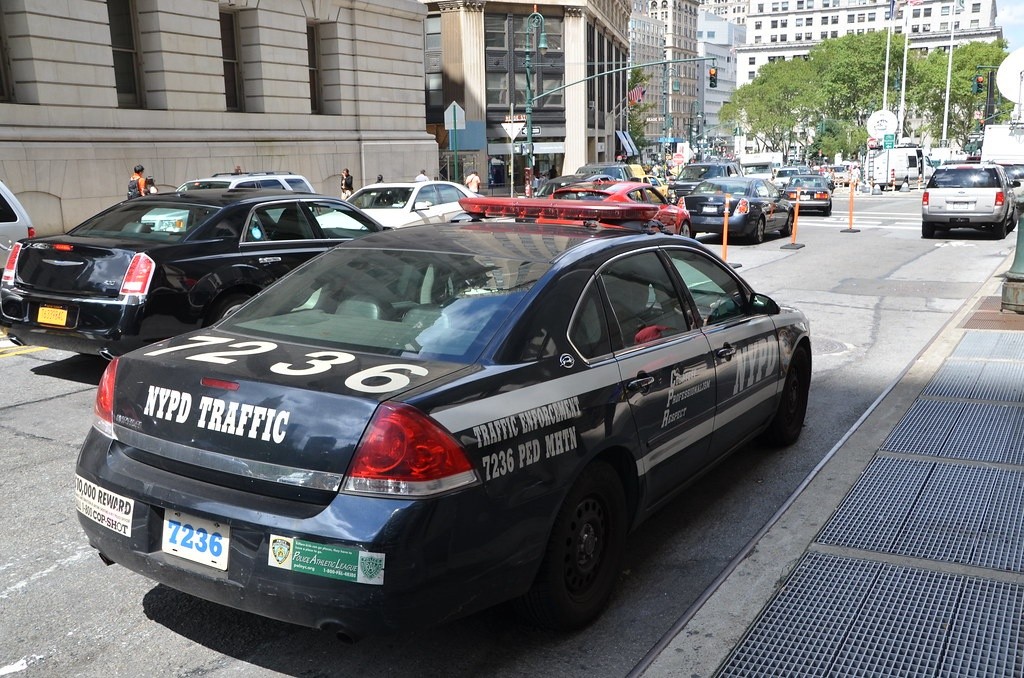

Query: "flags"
(629, 84), (645, 105)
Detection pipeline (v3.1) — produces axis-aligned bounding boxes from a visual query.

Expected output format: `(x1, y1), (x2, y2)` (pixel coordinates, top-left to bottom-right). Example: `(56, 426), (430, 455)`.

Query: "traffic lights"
(732, 127), (737, 136)
(973, 74), (983, 94)
(709, 68), (718, 87)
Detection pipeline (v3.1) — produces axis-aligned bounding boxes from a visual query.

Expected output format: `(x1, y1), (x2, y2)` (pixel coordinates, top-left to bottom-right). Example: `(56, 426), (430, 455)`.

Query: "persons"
(532, 165), (557, 196)
(341, 168), (353, 201)
(633, 324), (679, 345)
(852, 165), (861, 190)
(415, 169), (429, 181)
(618, 151), (627, 163)
(466, 171), (481, 194)
(788, 159), (794, 168)
(127, 165), (158, 200)
(376, 175), (384, 184)
(826, 169), (835, 197)
(650, 163), (674, 179)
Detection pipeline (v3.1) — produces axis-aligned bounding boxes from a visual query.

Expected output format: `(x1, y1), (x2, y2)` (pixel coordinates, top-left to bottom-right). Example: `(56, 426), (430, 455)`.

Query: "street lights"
(661, 68), (679, 161)
(524, 12), (549, 167)
(689, 100), (703, 148)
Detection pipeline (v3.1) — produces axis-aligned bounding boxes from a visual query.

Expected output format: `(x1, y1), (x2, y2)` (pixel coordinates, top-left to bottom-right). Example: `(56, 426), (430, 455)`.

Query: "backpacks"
(128, 177), (140, 199)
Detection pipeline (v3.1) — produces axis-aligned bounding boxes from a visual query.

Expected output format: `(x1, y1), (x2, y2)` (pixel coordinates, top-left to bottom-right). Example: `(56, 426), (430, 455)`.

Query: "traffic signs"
(883, 142), (894, 149)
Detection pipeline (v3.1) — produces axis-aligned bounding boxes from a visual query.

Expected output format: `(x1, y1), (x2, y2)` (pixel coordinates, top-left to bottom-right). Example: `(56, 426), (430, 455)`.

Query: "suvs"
(667, 160), (743, 204)
(172, 171), (322, 223)
(921, 161), (1024, 238)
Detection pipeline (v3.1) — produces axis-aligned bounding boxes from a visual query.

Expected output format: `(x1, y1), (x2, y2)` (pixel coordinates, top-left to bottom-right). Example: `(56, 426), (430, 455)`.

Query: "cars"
(449, 162), (691, 240)
(0, 188), (398, 362)
(71, 221), (813, 646)
(314, 180), (485, 230)
(675, 164), (849, 244)
(0, 177), (36, 274)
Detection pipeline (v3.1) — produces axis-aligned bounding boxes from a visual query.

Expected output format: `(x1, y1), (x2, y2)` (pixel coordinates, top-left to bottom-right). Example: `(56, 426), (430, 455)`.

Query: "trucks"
(740, 153), (783, 176)
(862, 143), (937, 190)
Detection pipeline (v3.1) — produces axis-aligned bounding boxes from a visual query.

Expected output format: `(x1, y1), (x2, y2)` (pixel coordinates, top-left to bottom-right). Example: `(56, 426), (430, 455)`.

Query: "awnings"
(615, 131), (638, 156)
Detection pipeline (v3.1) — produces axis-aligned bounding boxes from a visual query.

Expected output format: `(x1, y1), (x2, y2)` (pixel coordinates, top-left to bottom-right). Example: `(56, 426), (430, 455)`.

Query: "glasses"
(153, 180), (155, 182)
